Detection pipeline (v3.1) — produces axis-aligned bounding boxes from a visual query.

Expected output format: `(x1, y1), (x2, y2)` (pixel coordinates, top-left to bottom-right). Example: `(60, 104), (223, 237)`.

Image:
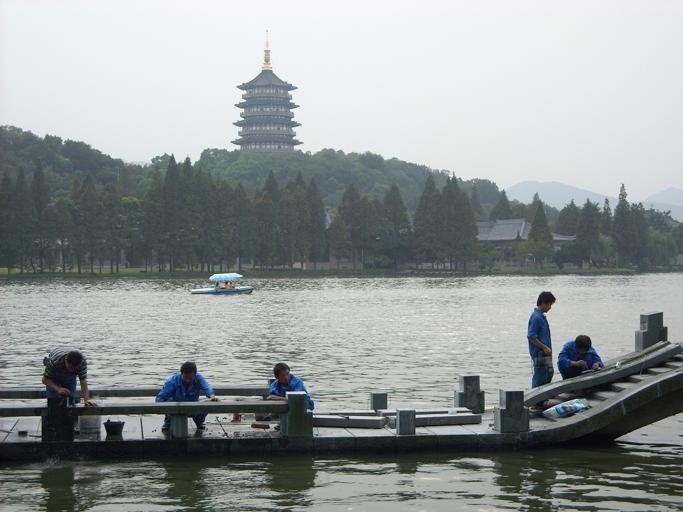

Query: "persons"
(527, 291), (555, 408)
(154, 361), (221, 430)
(222, 282), (229, 288)
(557, 335), (603, 380)
(228, 281), (234, 288)
(215, 282), (220, 289)
(267, 363), (314, 430)
(43, 347), (98, 429)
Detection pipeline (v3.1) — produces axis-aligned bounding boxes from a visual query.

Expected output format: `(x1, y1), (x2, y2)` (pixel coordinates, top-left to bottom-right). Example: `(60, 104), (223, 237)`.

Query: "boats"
(190, 271), (255, 295)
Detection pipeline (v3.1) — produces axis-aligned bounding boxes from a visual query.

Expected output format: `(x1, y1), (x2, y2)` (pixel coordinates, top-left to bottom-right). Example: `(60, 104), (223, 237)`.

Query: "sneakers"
(194, 417), (205, 429)
(162, 424), (167, 432)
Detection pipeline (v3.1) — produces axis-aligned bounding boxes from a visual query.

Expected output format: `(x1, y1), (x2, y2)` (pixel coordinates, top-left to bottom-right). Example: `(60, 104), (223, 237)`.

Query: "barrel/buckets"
(104, 416), (125, 436)
(75, 403), (102, 434)
